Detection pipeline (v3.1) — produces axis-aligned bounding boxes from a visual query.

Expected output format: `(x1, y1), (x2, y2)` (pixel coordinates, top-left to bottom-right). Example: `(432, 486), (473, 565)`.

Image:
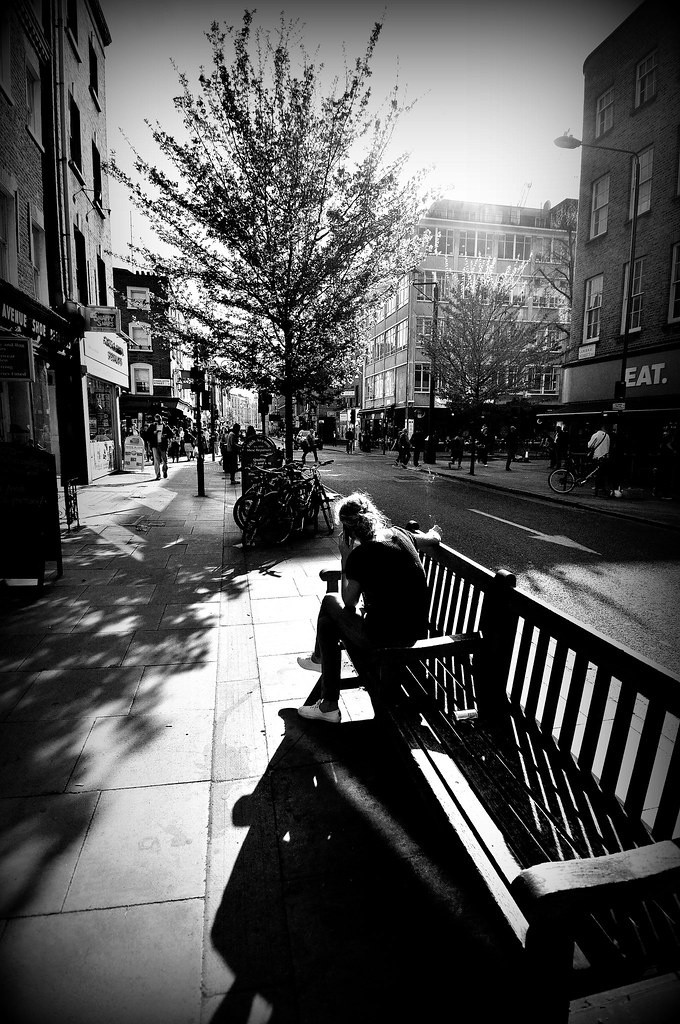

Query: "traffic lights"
(266, 392), (273, 414)
(201, 390), (211, 410)
(350, 409), (355, 425)
(212, 409), (219, 422)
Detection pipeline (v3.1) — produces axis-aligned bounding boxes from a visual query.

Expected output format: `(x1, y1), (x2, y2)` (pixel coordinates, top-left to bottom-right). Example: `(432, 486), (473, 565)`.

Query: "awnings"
(536, 395), (680, 419)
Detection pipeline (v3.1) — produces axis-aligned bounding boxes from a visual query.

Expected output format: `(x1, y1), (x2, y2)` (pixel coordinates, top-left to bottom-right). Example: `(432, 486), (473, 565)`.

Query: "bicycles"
(547, 454), (614, 499)
(232, 450), (335, 546)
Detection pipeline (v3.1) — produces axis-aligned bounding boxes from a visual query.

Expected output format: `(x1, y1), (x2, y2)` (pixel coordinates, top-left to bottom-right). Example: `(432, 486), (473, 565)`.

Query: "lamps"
(86, 207), (112, 222)
(73, 188), (103, 203)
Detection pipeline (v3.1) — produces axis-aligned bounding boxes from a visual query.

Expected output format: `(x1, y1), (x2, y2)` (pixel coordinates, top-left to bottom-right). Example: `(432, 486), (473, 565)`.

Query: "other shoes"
(231, 481), (240, 484)
(156, 475), (161, 480)
(163, 472), (167, 479)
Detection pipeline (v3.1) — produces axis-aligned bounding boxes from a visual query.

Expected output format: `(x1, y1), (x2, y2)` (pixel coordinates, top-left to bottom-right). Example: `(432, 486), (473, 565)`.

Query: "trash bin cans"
(238, 435), (278, 497)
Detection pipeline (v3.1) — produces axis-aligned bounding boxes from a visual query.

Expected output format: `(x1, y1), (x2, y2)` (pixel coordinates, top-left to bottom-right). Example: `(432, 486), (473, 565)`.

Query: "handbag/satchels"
(587, 449), (594, 459)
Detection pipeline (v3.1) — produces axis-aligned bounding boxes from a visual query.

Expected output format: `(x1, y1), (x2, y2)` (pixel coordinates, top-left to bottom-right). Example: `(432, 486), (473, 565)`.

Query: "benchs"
(318, 521), (680, 1024)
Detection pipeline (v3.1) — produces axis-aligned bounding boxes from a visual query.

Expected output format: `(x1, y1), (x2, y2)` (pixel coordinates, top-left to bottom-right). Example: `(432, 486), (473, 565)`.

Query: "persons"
(227, 423), (245, 485)
(660, 428), (678, 499)
(587, 424), (611, 491)
(147, 414), (175, 480)
(547, 426), (564, 470)
(331, 425), (498, 470)
(297, 492), (444, 723)
(121, 423), (230, 474)
(238, 425), (258, 472)
(300, 428), (321, 464)
(504, 425), (518, 472)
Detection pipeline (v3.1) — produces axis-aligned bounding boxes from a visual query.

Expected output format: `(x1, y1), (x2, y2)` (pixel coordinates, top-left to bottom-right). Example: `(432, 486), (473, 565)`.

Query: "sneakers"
(297, 653), (322, 673)
(298, 699), (341, 722)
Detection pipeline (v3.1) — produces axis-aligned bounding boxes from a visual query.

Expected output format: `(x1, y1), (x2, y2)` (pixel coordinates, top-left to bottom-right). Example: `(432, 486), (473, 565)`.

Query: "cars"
(296, 430), (324, 452)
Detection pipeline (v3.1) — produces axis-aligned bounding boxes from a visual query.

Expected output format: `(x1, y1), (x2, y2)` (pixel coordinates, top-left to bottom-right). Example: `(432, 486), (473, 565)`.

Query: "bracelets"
(340, 568), (346, 575)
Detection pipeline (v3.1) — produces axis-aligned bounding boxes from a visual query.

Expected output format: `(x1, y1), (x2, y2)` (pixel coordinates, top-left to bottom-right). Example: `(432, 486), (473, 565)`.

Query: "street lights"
(553, 135), (641, 485)
